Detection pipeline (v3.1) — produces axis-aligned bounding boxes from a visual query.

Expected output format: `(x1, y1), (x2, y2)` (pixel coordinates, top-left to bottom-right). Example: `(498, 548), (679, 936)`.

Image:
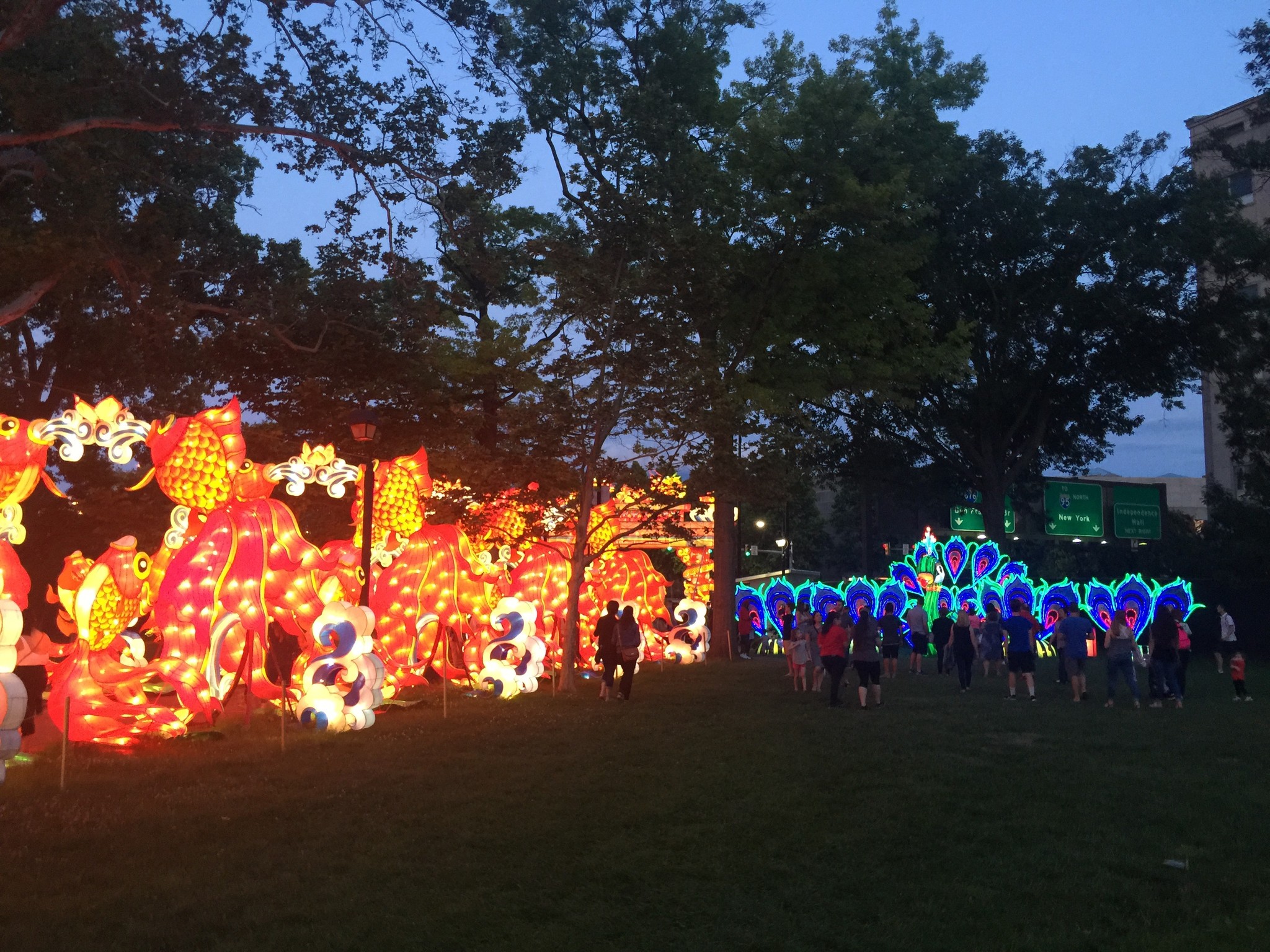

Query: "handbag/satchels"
(621, 648), (639, 661)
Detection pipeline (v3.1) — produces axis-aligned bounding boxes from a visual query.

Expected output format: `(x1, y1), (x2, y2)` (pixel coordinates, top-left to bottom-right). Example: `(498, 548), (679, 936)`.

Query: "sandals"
(617, 693), (623, 704)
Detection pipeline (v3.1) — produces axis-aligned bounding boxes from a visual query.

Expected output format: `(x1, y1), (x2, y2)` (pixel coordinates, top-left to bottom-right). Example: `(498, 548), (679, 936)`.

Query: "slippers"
(599, 694), (605, 699)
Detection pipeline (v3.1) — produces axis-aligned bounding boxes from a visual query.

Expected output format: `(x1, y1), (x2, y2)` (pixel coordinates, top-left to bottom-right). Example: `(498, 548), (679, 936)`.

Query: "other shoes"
(1082, 691), (1089, 699)
(875, 702), (885, 708)
(1103, 701), (1114, 707)
(861, 704), (872, 711)
(1073, 695), (1081, 702)
(740, 653), (747, 659)
(909, 668), (916, 673)
(1134, 701), (1141, 708)
(745, 653), (751, 660)
(1149, 703), (1162, 708)
(916, 671), (928, 676)
(828, 701), (845, 709)
(1219, 669), (1224, 674)
(1176, 702), (1183, 709)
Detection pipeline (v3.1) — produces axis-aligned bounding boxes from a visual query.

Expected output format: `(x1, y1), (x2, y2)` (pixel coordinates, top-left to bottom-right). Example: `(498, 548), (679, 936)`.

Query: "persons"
(611, 606), (640, 703)
(593, 601), (620, 703)
(1199, 603), (1243, 674)
(658, 598), (713, 645)
(738, 599), (755, 660)
(774, 597), (1195, 710)
(1229, 644), (1254, 703)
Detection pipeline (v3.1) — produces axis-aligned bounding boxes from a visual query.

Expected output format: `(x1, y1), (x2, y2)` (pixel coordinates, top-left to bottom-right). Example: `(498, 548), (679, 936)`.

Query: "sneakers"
(1234, 696), (1242, 701)
(1030, 696), (1036, 701)
(1245, 697), (1253, 702)
(1004, 694), (1016, 700)
